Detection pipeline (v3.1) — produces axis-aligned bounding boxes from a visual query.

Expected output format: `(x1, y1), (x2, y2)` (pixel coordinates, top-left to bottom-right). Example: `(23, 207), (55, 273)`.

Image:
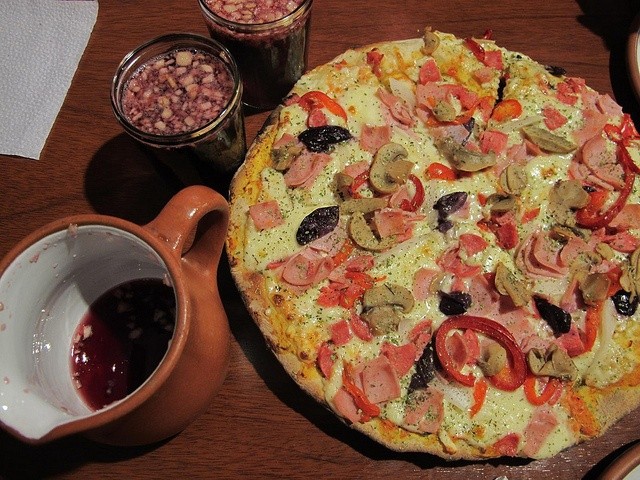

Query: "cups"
(109, 30), (248, 193)
(0, 183), (232, 451)
(196, 0), (315, 111)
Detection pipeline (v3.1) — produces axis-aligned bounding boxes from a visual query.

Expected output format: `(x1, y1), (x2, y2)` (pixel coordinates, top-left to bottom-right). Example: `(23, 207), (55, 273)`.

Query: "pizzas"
(224, 25), (640, 462)
